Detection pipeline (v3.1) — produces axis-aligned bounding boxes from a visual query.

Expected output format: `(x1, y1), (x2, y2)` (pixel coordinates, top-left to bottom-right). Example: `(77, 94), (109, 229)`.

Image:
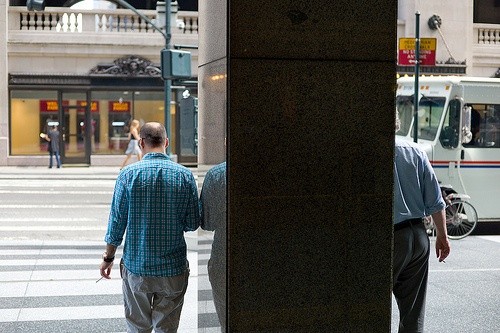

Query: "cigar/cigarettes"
(96, 276), (104, 282)
(437, 257), (446, 264)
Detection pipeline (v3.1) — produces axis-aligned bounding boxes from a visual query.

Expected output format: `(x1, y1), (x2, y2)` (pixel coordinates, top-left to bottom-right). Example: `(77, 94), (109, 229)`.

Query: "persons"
(393, 106), (450, 333)
(119, 120), (142, 171)
(100, 121), (202, 333)
(465, 104), (483, 145)
(200, 137), (226, 333)
(45, 125), (64, 169)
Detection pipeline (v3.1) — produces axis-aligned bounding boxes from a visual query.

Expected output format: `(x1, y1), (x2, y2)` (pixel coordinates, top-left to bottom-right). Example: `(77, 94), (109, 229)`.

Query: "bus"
(396, 76), (499, 222)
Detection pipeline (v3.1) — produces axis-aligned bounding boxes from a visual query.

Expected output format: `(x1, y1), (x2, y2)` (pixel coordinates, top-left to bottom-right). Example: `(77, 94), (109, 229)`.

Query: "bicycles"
(423, 180), (479, 240)
